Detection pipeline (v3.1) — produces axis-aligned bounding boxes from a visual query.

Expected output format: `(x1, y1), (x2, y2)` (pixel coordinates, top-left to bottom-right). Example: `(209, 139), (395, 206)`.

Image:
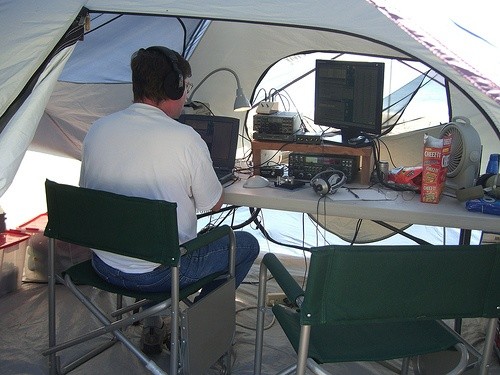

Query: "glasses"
(185, 81), (193, 93)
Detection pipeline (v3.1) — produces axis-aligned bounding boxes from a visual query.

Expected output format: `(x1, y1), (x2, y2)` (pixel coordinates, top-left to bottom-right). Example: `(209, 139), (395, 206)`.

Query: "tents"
(0, 0), (499, 205)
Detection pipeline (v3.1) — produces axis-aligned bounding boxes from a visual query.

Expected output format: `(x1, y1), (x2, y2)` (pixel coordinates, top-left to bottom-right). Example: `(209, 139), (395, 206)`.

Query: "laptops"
(174, 114), (240, 185)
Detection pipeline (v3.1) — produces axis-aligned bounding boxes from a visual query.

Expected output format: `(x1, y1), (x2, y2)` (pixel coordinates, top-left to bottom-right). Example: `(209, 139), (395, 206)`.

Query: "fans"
(439, 116), (483, 198)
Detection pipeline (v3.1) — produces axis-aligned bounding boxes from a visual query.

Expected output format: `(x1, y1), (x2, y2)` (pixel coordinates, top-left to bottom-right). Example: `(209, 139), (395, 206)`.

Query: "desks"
(251, 138), (376, 185)
(218, 162), (500, 342)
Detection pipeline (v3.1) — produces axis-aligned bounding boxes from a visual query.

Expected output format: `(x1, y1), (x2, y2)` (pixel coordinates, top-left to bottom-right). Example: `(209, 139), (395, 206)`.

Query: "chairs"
(254, 242), (500, 375)
(44, 179), (237, 375)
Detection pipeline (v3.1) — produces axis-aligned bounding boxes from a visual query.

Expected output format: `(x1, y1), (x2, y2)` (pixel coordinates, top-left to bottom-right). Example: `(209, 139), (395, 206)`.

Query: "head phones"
(147, 46), (185, 100)
(310, 170), (346, 196)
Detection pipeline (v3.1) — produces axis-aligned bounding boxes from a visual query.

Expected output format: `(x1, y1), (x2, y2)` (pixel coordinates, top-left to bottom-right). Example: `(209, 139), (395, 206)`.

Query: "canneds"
(379, 162), (389, 183)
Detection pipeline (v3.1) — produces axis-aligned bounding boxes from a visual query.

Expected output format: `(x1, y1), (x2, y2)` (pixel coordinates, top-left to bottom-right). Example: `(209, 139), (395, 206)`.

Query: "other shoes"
(140, 320), (181, 355)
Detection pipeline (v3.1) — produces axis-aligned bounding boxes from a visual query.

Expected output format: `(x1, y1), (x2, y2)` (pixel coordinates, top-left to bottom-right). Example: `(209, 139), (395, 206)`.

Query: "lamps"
(185, 67), (253, 112)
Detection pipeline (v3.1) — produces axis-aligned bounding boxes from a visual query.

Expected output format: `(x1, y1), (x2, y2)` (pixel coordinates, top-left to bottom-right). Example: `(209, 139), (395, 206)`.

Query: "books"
(326, 187), (390, 201)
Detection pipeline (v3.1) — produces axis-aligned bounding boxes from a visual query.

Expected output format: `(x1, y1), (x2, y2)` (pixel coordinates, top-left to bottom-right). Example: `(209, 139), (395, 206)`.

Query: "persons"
(79, 46), (260, 354)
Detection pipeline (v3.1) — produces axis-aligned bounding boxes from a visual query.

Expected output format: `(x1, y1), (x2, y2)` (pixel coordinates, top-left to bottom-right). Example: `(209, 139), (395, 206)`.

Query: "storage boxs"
(16, 210), (92, 281)
(422, 136), (452, 203)
(0, 230), (31, 296)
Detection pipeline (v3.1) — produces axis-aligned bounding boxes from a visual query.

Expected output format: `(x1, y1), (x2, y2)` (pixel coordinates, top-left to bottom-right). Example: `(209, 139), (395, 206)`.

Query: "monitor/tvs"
(315, 60), (385, 148)
